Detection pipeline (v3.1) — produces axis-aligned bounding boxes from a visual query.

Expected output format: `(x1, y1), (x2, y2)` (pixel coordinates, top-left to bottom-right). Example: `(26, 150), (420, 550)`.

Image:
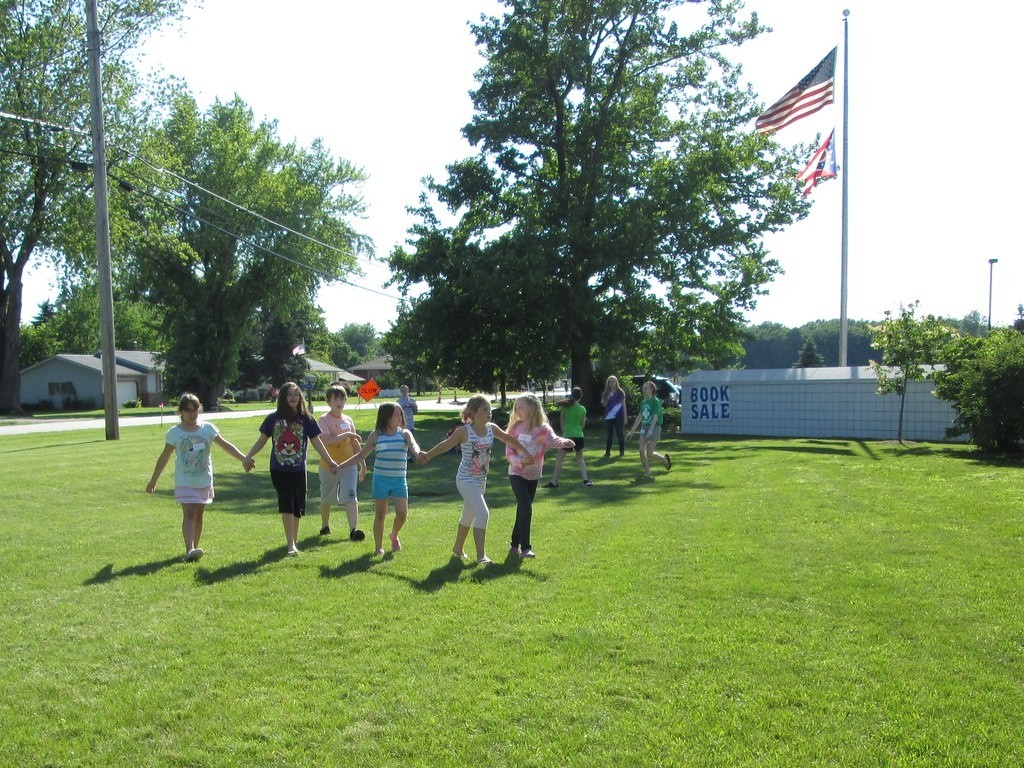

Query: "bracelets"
(340, 464), (344, 468)
(406, 404), (409, 408)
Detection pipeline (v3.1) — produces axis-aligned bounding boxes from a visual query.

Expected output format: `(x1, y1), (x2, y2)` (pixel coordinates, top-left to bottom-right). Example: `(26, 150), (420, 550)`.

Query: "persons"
(505, 392), (577, 558)
(145, 394), (256, 565)
(317, 386), (365, 540)
(396, 384), (419, 464)
(420, 396), (534, 565)
(626, 381), (672, 477)
(541, 387), (593, 488)
(601, 376), (628, 458)
(334, 402), (426, 558)
(241, 382), (338, 556)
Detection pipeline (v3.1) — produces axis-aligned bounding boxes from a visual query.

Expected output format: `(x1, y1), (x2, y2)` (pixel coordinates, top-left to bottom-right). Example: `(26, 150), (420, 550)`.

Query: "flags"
(754, 45), (837, 138)
(293, 344), (304, 356)
(795, 128), (838, 198)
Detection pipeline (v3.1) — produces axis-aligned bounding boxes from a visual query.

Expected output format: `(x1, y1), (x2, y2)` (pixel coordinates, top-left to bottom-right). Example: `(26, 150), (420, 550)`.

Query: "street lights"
(987, 257), (998, 330)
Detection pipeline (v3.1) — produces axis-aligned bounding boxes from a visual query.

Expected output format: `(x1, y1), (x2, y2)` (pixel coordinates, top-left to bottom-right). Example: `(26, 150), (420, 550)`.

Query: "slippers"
(663, 453), (671, 470)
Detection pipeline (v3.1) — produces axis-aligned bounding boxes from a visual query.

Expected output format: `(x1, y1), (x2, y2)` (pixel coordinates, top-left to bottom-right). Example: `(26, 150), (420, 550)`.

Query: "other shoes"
(541, 482), (559, 487)
(583, 479), (594, 486)
(510, 546), (535, 558)
(389, 532), (401, 551)
(376, 547), (384, 554)
(183, 548), (203, 561)
(452, 546), (468, 558)
(320, 527), (331, 535)
(350, 528), (365, 541)
(287, 548), (300, 557)
(477, 555), (492, 564)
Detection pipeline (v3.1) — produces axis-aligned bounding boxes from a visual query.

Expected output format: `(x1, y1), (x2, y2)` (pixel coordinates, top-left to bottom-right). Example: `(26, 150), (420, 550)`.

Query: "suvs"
(628, 376), (680, 404)
